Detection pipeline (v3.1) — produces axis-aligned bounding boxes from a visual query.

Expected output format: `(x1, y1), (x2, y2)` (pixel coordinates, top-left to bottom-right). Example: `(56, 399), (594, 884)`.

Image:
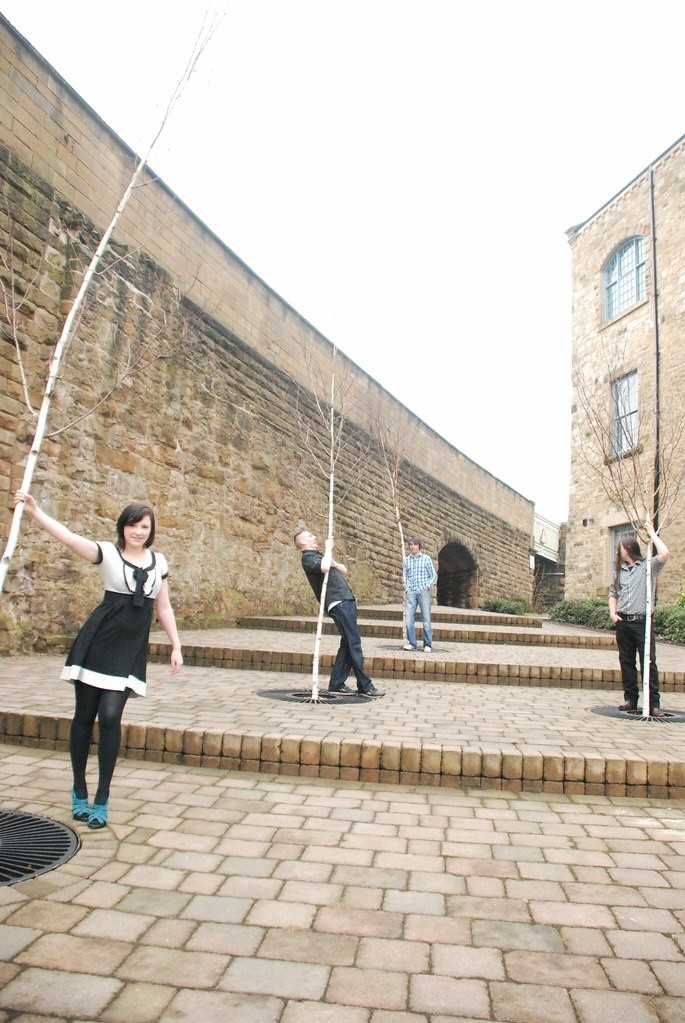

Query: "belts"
(620, 615), (645, 622)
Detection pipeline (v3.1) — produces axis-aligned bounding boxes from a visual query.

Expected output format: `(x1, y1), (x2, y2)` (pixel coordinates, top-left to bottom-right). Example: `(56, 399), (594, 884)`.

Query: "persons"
(402, 539), (437, 652)
(609, 520), (669, 718)
(13, 489), (183, 829)
(293, 531), (385, 699)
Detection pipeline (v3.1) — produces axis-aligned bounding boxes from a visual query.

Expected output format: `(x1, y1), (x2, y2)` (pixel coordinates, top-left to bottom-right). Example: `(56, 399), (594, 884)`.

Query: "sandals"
(72, 787), (90, 821)
(87, 794), (111, 828)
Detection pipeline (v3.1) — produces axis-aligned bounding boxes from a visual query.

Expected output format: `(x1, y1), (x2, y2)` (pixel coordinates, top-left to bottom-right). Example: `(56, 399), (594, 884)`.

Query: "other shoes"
(403, 644), (416, 650)
(619, 701), (637, 711)
(424, 646), (432, 652)
(650, 707), (666, 718)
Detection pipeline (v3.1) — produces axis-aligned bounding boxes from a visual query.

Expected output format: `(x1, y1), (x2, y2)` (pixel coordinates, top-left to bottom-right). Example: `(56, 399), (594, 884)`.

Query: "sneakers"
(357, 685), (385, 698)
(328, 683), (355, 695)
